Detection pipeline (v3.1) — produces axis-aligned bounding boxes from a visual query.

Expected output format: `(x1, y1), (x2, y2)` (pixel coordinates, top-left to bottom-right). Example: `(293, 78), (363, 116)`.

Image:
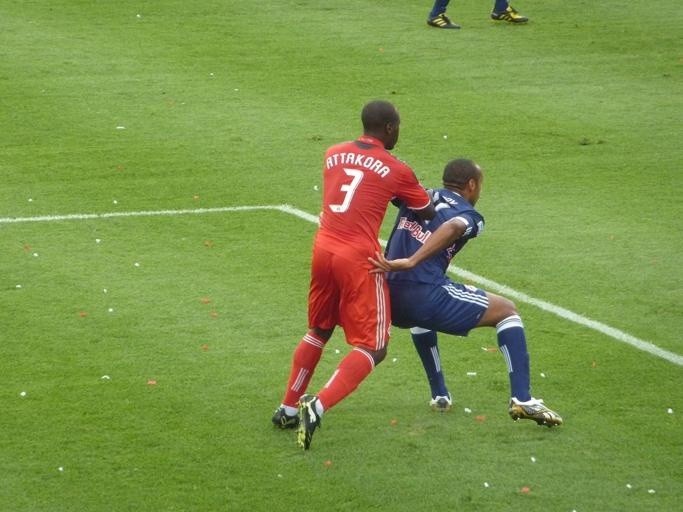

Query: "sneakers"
(427, 14), (461, 30)
(430, 393), (452, 412)
(271, 407), (300, 429)
(295, 393), (321, 450)
(491, 6), (529, 23)
(509, 397), (564, 428)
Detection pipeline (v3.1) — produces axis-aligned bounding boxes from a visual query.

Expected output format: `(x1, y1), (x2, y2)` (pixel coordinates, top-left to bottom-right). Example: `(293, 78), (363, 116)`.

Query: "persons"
(269, 99), (437, 455)
(424, 2), (531, 31)
(361, 156), (565, 427)
